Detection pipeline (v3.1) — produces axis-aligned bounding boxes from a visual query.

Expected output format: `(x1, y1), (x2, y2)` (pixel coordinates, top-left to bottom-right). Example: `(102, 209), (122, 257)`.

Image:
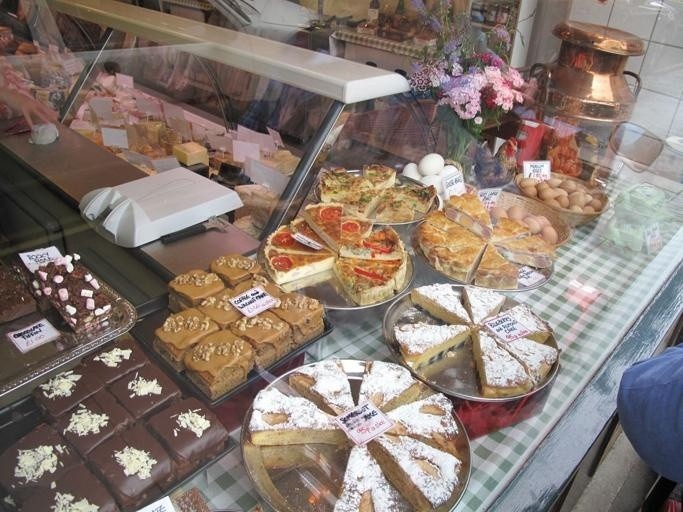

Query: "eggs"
(520, 177), (602, 214)
(490, 205), (560, 242)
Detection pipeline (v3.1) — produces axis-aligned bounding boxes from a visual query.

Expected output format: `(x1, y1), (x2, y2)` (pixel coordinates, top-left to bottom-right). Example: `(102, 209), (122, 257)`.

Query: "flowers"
(406, 0), (538, 171)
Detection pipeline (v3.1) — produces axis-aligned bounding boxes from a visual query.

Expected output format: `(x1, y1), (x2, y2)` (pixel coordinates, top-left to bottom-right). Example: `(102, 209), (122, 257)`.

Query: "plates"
(410, 211), (556, 291)
(383, 283), (561, 403)
(262, 219), (417, 313)
(312, 169), (443, 227)
(239, 358), (473, 512)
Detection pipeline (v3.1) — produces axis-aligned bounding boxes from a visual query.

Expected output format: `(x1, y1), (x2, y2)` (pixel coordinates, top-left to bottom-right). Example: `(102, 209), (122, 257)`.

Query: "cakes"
(263, 161), (556, 306)
(248, 283), (558, 512)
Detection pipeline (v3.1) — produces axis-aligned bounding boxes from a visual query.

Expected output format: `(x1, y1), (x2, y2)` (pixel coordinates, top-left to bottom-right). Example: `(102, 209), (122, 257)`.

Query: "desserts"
(0, 251), (325, 512)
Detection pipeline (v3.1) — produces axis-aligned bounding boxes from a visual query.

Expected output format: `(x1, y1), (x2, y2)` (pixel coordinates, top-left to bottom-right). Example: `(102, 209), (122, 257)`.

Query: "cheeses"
(1, 27), (302, 239)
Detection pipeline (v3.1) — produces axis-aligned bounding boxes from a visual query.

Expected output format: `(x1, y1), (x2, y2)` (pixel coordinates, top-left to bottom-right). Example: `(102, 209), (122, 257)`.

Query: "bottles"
(529, 20), (646, 190)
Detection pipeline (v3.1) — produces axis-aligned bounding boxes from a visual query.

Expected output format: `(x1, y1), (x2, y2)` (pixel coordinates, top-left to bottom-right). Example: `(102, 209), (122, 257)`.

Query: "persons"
(291, 95), (356, 153)
(617, 341), (682, 486)
(0, 88), (59, 133)
(239, 85), (314, 146)
